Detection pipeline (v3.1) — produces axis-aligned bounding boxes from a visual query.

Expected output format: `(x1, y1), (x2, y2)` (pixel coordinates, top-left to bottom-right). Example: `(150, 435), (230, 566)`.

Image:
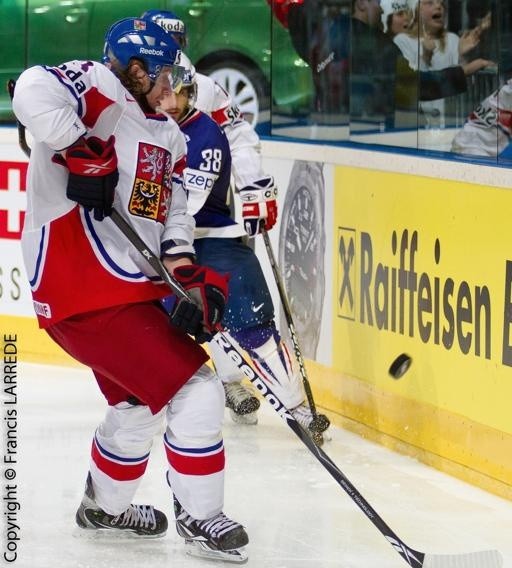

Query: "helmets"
(104, 9), (199, 112)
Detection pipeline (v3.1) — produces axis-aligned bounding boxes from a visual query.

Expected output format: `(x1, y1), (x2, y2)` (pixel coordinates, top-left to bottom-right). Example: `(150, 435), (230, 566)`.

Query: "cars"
(0, 0), (317, 158)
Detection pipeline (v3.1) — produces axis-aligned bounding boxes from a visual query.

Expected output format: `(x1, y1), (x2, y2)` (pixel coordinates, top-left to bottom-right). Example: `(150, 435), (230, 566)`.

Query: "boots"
(172, 495), (249, 551)
(283, 407), (330, 434)
(73, 503), (169, 536)
(219, 381), (260, 415)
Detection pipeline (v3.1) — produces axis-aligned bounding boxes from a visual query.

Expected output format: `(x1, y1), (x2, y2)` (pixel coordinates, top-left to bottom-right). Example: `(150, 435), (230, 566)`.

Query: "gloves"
(51, 131), (120, 222)
(238, 177), (280, 235)
(169, 264), (230, 345)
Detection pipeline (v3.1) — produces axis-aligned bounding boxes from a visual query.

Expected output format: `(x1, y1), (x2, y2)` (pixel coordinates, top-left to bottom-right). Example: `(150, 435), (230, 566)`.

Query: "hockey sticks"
(258, 220), (325, 445)
(51, 147), (503, 568)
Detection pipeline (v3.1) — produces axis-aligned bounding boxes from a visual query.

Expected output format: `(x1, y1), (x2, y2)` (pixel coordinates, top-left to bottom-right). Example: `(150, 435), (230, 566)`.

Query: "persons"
(312, 0), (496, 128)
(12, 11), (330, 551)
